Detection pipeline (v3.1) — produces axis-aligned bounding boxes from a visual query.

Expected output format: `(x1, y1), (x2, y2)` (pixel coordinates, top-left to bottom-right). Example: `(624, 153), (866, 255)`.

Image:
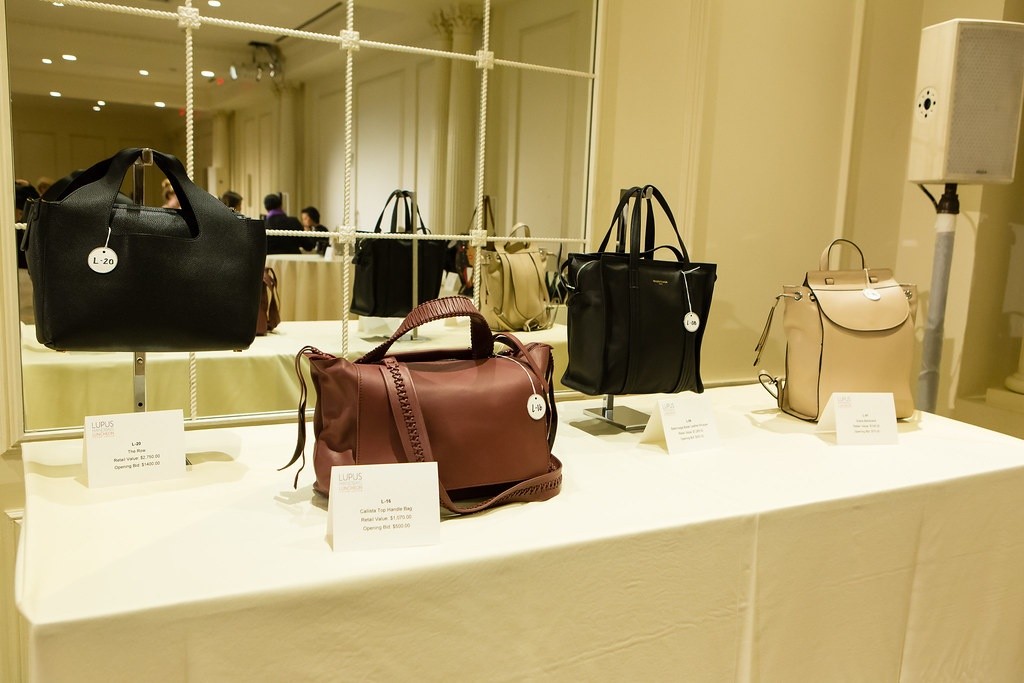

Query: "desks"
(265, 253), (356, 322)
(5, 378), (1023, 683)
(20, 318), (568, 432)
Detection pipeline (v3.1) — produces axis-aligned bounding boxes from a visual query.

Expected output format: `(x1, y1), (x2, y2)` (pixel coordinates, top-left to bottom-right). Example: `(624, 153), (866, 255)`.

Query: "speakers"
(908, 18), (1024, 185)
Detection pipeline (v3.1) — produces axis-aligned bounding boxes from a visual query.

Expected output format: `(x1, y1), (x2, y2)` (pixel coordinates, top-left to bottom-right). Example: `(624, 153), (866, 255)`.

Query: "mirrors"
(0, 0), (607, 458)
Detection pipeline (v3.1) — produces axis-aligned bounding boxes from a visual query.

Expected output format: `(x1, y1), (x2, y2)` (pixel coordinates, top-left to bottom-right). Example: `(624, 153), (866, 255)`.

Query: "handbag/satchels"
(20, 146), (265, 351)
(458, 202), (497, 268)
(562, 184), (717, 395)
(275, 295), (563, 516)
(544, 269), (569, 304)
(475, 223), (560, 330)
(752, 237), (920, 423)
(259, 265), (282, 337)
(460, 266), (477, 298)
(348, 189), (452, 319)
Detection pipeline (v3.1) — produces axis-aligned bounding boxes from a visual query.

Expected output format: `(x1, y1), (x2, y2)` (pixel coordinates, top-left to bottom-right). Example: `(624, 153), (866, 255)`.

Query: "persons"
(264, 194), (305, 254)
(301, 207), (330, 256)
(222, 191), (242, 213)
(161, 178), (181, 209)
